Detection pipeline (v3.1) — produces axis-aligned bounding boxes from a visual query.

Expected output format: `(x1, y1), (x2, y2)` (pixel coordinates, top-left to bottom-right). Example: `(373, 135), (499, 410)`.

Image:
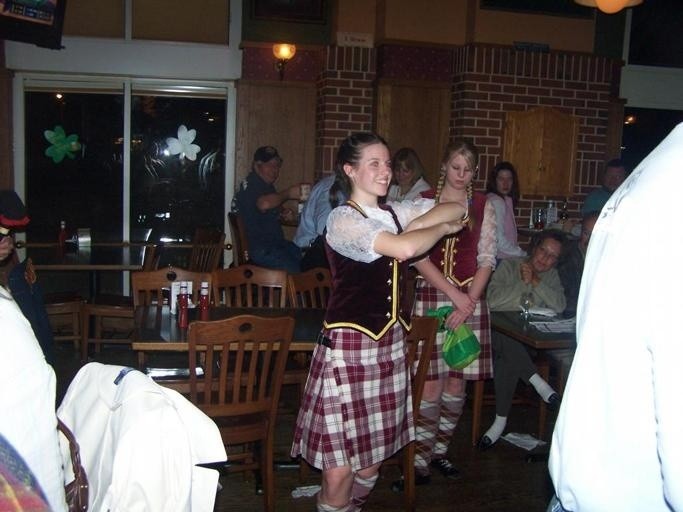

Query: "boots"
(528, 373), (558, 403)
(481, 415), (507, 446)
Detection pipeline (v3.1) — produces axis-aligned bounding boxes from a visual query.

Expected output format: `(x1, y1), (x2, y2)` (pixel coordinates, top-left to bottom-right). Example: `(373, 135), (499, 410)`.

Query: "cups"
(298, 183), (312, 203)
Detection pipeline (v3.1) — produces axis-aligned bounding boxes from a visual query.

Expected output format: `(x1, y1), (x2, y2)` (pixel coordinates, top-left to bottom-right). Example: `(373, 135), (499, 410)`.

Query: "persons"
(233, 133), (629, 512)
(0, 189), (70, 512)
(547, 122), (683, 512)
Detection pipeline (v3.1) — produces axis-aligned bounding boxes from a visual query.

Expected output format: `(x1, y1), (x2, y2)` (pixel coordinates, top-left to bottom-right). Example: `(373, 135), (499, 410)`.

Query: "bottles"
(199, 281), (209, 322)
(58, 220), (70, 247)
(177, 281), (189, 328)
(535, 199), (570, 230)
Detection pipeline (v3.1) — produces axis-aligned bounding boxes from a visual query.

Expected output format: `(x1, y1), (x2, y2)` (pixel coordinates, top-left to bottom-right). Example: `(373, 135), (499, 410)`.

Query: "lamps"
(273, 43), (296, 81)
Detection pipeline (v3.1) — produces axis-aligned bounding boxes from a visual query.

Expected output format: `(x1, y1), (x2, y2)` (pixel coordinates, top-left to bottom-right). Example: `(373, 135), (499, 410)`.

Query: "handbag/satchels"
(428, 307), (482, 370)
(65, 479), (88, 512)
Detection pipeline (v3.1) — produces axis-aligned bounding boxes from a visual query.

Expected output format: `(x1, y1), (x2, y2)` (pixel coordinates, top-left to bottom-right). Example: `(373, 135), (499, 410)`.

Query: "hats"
(254, 146), (283, 163)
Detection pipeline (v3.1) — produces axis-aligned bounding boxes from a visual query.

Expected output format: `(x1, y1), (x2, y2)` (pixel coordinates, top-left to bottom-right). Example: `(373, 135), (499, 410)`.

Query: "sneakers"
(439, 459), (457, 476)
(395, 466), (431, 491)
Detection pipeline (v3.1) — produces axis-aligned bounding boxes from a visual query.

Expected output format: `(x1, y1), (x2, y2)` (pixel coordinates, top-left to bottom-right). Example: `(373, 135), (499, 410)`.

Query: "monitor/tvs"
(0, 0), (67, 50)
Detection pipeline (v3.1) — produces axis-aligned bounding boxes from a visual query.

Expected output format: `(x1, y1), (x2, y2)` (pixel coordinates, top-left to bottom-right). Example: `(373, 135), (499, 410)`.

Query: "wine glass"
(518, 291), (537, 323)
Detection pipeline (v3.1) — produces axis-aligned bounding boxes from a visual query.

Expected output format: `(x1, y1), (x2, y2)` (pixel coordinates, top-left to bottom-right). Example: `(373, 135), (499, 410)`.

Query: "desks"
(472, 312), (576, 447)
(27, 245), (146, 361)
